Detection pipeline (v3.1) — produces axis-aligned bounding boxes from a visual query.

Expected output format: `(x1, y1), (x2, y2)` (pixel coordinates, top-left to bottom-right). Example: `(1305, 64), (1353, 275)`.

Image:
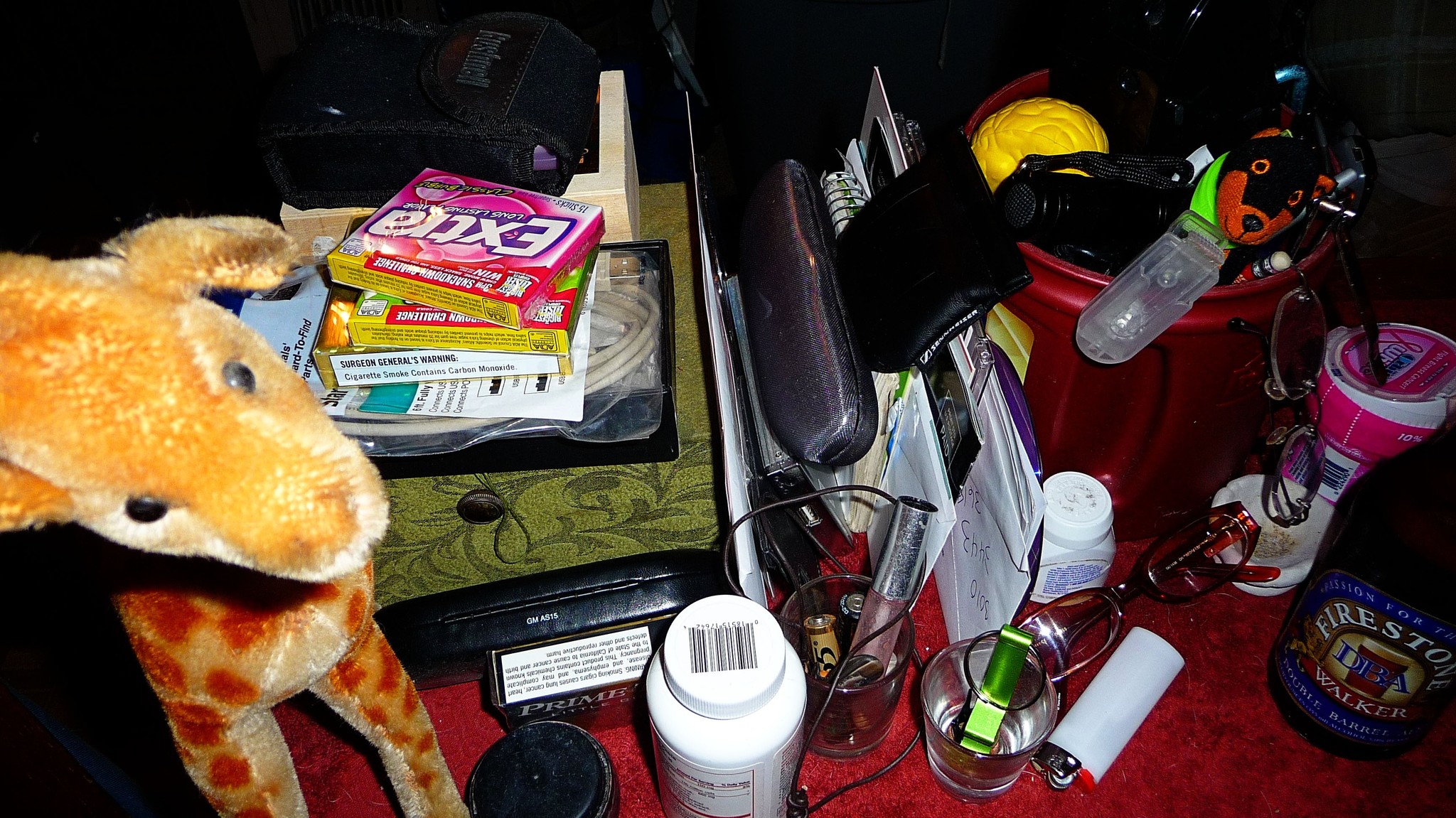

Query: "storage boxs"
(312, 214), (572, 388)
(350, 246), (602, 354)
(328, 168), (604, 327)
(486, 609), (675, 734)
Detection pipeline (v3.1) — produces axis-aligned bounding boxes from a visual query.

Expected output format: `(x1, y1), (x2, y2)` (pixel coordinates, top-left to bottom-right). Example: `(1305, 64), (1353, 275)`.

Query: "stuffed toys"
(0, 216), (473, 818)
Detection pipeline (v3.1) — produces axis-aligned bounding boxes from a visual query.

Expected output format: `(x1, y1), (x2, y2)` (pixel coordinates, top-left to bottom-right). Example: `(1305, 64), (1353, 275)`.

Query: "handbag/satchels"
(259, 8), (602, 207)
(992, 150), (1195, 288)
(829, 124), (1032, 373)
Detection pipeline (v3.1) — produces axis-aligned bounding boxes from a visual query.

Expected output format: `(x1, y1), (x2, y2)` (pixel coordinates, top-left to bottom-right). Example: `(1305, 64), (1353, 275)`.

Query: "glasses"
(1018, 498), (1285, 683)
(1227, 286), (1328, 525)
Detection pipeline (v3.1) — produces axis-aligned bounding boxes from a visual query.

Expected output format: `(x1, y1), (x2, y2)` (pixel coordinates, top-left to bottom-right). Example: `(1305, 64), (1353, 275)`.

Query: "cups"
(778, 574), (916, 758)
(920, 637), (1059, 803)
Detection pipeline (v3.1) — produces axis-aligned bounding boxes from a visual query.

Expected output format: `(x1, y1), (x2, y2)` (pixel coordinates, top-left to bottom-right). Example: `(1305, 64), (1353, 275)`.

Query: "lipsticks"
(847, 497), (939, 678)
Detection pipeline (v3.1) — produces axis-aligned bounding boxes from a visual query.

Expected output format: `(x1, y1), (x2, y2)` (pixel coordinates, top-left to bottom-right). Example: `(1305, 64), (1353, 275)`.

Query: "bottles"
(1028, 471), (1116, 606)
(1280, 322), (1456, 509)
(1264, 410), (1456, 763)
(646, 593), (808, 818)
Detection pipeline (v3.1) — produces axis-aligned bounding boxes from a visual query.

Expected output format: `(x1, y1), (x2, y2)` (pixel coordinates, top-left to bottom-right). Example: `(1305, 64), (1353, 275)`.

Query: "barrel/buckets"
(962, 67), (1346, 537)
